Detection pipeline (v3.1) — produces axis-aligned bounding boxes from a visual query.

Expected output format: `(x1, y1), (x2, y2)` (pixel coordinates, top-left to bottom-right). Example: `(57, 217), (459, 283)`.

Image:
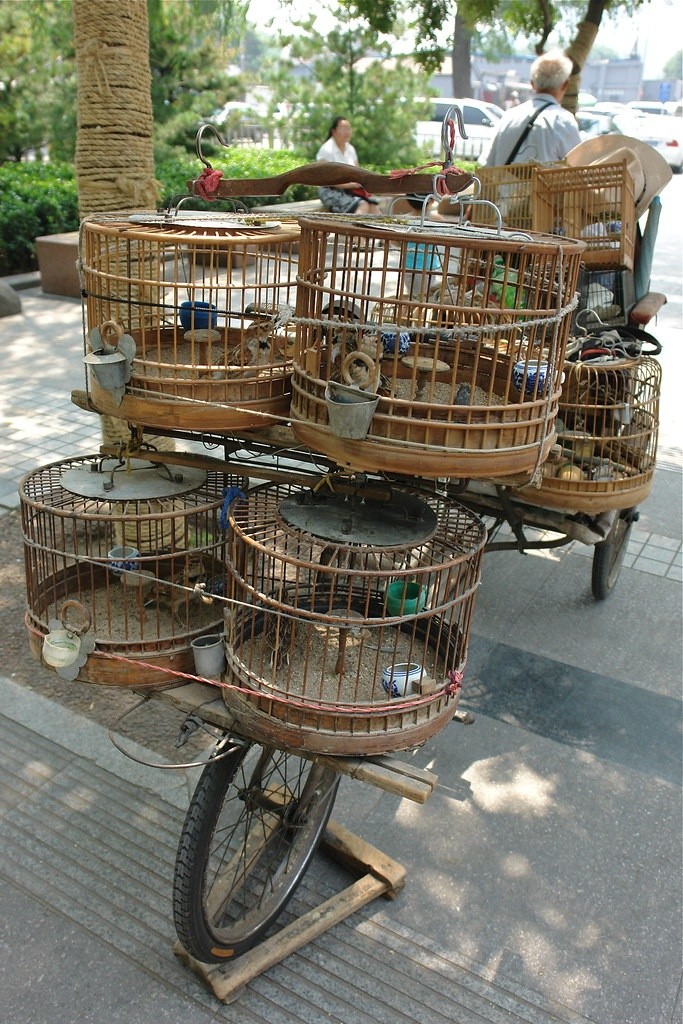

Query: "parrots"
(328, 343), (401, 398)
(200, 318), (281, 378)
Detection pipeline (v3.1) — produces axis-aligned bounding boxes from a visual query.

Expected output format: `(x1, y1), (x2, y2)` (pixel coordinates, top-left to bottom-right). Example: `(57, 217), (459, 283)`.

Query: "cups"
(375, 323), (410, 359)
(109, 547), (139, 577)
(385, 580), (425, 615)
(514, 359), (550, 395)
(179, 302), (218, 329)
(382, 662), (425, 698)
(191, 634), (226, 679)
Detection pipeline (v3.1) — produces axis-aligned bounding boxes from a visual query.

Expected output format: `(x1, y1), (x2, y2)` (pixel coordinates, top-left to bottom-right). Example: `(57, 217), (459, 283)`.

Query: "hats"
(562, 135), (674, 222)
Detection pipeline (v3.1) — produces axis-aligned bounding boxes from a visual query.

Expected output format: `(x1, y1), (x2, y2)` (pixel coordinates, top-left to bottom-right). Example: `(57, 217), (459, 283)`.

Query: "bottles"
(493, 255), (532, 318)
(469, 277), (496, 303)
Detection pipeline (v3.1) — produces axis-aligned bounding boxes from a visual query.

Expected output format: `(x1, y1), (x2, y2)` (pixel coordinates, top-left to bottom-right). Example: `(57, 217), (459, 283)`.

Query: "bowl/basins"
(606, 219), (622, 234)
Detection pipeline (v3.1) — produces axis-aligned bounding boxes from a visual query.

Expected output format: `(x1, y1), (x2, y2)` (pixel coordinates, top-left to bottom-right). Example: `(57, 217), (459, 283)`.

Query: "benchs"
(251, 194), (412, 253)
(35, 231), (258, 300)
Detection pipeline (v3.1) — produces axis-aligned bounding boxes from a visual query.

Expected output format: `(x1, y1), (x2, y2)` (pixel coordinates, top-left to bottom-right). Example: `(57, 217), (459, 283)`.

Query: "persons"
(315, 117), (390, 250)
(506, 91), (520, 110)
(481, 48), (583, 227)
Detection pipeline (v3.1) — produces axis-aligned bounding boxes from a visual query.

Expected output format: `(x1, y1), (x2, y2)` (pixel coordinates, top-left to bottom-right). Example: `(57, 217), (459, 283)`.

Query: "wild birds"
(581, 208), (622, 223)
(433, 289), (522, 335)
(140, 561), (215, 629)
(265, 590), (301, 670)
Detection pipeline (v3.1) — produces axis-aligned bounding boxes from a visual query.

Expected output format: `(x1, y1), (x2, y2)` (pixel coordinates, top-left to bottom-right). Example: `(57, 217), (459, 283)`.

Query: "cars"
(195, 81), (683, 176)
